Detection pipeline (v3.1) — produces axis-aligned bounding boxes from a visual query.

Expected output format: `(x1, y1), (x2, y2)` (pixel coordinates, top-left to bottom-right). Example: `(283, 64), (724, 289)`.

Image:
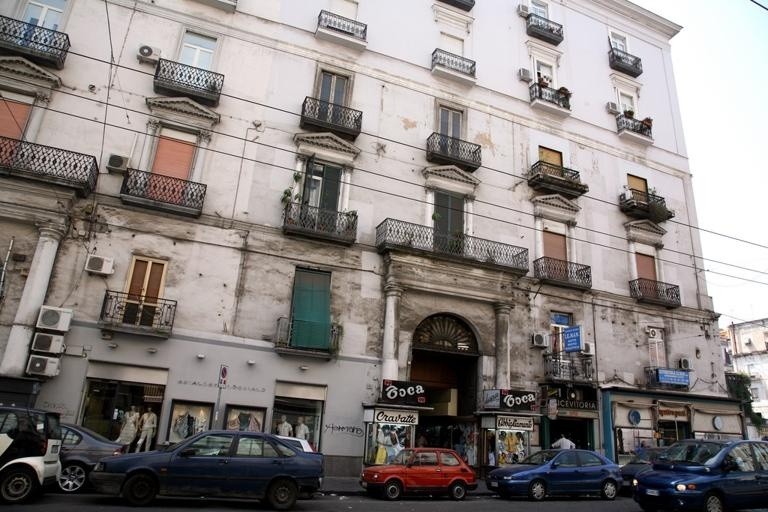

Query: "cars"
(91, 429), (323, 509)
(360, 446), (478, 498)
(37, 422), (129, 492)
(486, 448), (622, 500)
(0, 404), (63, 504)
(621, 438), (768, 512)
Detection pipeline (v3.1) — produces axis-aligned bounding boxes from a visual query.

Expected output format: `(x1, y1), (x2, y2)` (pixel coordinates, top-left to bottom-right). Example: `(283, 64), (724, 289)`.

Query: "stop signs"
(218, 365), (229, 388)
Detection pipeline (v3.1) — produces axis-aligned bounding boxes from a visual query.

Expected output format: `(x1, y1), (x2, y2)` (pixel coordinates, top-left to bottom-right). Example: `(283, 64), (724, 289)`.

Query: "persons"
(515, 432), (525, 458)
(276, 414), (293, 438)
(135, 406), (157, 454)
(195, 409), (207, 434)
(113, 403), (139, 455)
(295, 417), (310, 442)
(550, 432), (575, 449)
(374, 425), (386, 464)
(381, 426), (404, 458)
(498, 431), (508, 464)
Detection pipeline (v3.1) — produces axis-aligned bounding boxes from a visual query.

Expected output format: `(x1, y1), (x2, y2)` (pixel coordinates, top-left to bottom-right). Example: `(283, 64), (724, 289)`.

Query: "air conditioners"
(82, 254), (116, 277)
(581, 342), (596, 357)
(607, 102), (621, 116)
(137, 44), (161, 64)
(619, 190), (632, 202)
(649, 329), (661, 339)
(529, 332), (550, 349)
(517, 3), (529, 17)
(680, 357), (695, 370)
(106, 153), (130, 174)
(26, 305), (74, 379)
(519, 68), (533, 83)
